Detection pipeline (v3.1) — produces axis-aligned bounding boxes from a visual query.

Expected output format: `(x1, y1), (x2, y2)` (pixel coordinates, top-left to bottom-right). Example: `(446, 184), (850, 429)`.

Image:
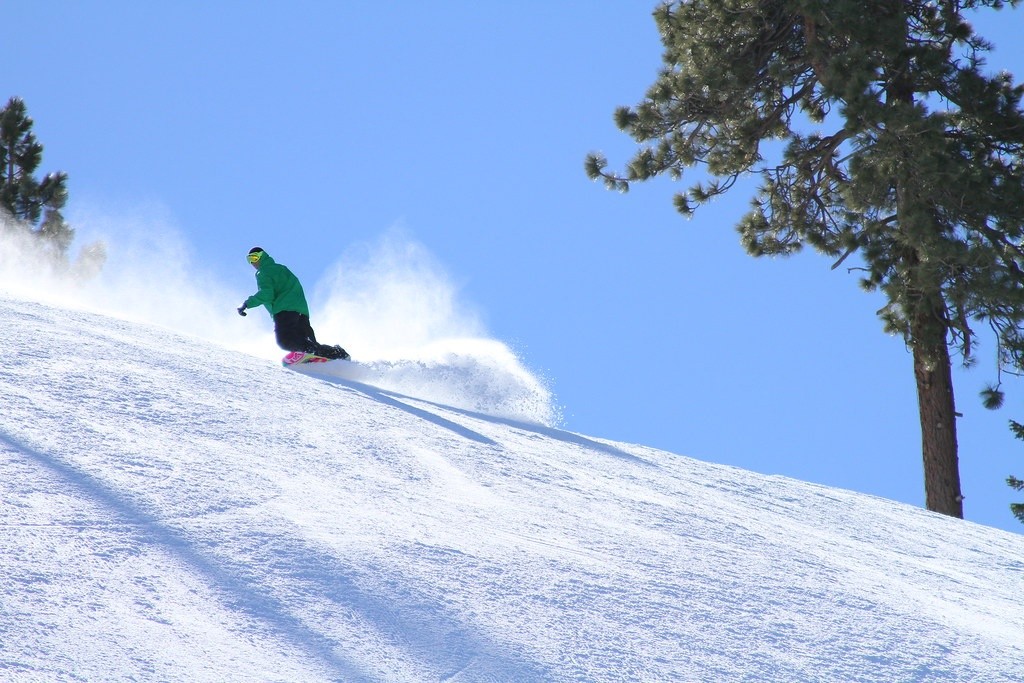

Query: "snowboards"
(281, 352), (332, 366)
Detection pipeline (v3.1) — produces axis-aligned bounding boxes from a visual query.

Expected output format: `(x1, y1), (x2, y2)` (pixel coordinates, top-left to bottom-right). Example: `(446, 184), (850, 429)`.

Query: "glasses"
(245, 251), (263, 264)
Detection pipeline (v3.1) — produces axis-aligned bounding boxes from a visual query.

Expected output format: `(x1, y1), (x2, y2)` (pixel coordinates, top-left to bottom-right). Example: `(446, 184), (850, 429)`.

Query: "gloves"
(237, 302), (247, 316)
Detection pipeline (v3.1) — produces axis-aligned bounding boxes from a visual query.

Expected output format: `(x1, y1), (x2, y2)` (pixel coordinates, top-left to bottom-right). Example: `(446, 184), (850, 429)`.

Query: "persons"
(237, 247), (352, 360)
